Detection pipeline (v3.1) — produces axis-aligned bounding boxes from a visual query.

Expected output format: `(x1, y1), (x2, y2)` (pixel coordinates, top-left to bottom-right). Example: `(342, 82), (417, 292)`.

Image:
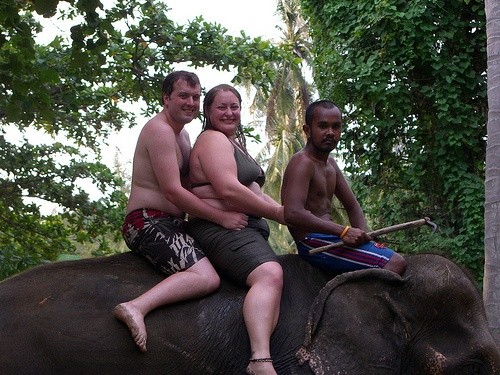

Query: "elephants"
(0, 250), (500, 374)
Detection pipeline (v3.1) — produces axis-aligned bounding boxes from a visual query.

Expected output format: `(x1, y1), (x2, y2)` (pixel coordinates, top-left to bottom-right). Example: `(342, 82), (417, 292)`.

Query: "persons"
(187, 83), (296, 375)
(279, 100), (407, 275)
(112, 71), (248, 353)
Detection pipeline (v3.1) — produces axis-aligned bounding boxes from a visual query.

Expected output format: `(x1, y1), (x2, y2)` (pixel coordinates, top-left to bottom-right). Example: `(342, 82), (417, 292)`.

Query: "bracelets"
(339, 225), (350, 239)
